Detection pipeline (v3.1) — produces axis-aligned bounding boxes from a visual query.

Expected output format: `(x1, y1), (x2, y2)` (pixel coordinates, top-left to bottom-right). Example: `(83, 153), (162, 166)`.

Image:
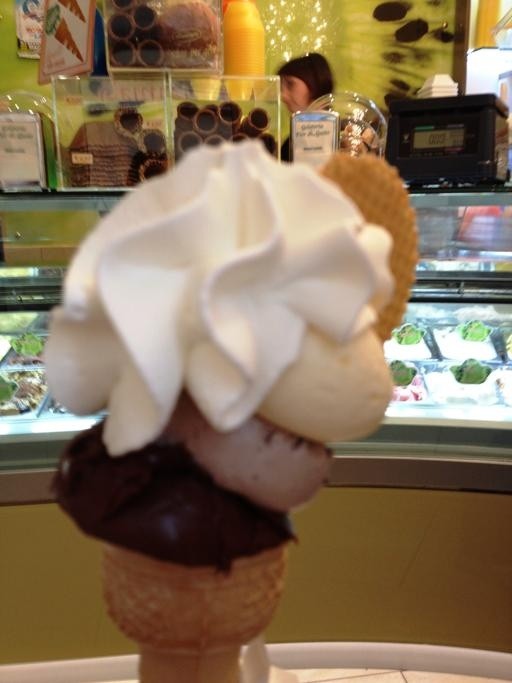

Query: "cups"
(223, 0), (265, 101)
(190, 79), (221, 101)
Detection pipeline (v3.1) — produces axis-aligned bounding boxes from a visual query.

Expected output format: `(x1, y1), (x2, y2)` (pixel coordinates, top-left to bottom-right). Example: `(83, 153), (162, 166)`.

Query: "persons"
(277, 52), (333, 162)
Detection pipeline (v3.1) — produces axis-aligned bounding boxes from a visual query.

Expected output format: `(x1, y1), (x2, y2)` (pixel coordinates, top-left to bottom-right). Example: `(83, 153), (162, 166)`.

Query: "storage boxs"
(103, 0), (219, 74)
(168, 73), (281, 168)
(53, 75), (168, 191)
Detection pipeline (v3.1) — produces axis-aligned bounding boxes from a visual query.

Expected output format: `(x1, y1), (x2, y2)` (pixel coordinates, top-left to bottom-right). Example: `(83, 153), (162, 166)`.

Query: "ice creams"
(42, 5), (83, 62)
(40, 138), (419, 682)
(60, 1), (86, 23)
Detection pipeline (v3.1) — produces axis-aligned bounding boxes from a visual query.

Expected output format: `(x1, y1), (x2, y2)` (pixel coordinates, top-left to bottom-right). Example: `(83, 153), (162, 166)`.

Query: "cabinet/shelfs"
(0, 189), (512, 505)
